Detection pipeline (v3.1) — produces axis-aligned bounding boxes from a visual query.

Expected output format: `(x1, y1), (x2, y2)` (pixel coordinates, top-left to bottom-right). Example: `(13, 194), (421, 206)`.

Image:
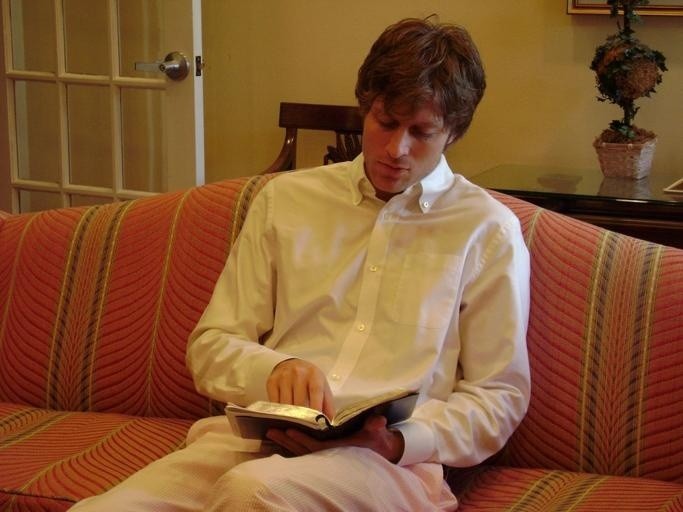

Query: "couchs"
(0, 168), (683, 512)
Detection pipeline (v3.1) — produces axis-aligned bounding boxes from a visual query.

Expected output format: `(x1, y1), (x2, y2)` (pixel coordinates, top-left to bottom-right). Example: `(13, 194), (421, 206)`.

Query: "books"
(223, 386), (420, 438)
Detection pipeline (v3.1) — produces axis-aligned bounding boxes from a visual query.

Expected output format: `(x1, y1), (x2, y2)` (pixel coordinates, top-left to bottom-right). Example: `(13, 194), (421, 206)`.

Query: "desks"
(467, 164), (683, 248)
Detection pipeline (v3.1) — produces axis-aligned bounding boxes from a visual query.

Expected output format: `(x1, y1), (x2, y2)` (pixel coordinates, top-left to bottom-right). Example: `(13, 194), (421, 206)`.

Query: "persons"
(68, 19), (532, 512)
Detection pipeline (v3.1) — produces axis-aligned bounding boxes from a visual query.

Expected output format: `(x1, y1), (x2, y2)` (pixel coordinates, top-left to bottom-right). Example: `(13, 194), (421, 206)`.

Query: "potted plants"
(592, 0), (668, 179)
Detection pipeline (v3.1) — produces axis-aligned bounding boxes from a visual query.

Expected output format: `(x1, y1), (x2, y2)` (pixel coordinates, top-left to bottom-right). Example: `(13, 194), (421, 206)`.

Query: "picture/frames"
(567, 0), (683, 16)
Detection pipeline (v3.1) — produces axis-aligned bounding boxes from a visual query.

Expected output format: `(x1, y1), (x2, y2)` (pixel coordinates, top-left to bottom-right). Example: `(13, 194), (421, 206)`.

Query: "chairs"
(257, 101), (364, 174)
